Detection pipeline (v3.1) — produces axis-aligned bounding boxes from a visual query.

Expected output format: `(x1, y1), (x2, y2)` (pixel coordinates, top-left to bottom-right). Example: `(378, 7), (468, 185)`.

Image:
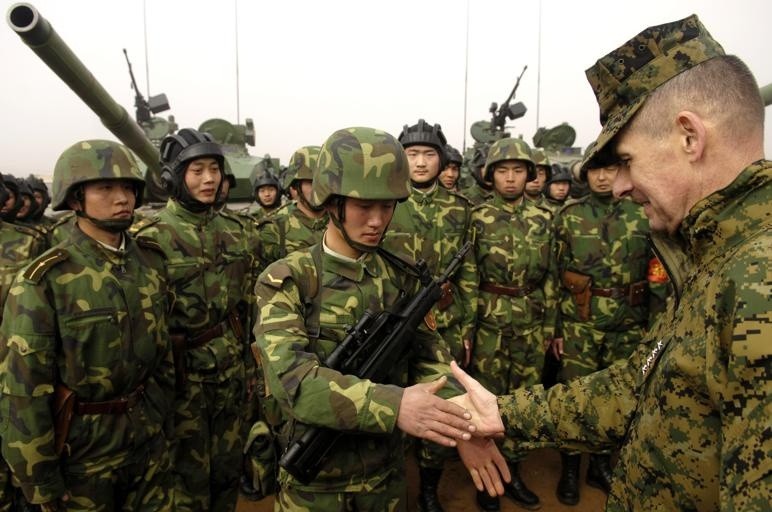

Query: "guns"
(279, 239), (477, 487)
(473, 65), (528, 169)
(123, 48), (170, 128)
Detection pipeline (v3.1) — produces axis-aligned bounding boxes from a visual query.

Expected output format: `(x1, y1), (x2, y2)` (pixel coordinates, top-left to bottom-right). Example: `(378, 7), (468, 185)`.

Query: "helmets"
(484, 138), (537, 182)
(52, 140), (145, 210)
(546, 163), (573, 192)
(446, 144), (463, 166)
(253, 171), (281, 198)
(473, 146), (491, 167)
(531, 149), (552, 182)
(397, 119), (447, 157)
(281, 146), (322, 189)
(158, 128), (237, 198)
(0, 173), (50, 218)
(312, 126), (412, 207)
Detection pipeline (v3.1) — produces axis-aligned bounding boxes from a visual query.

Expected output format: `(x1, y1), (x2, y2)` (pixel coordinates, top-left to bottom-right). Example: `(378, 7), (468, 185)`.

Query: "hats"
(581, 14), (725, 173)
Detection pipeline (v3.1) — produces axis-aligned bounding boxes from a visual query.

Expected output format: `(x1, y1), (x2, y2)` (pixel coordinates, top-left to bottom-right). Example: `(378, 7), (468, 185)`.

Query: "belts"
(77, 384), (146, 414)
(483, 285), (535, 296)
(187, 320), (229, 347)
(593, 288), (628, 297)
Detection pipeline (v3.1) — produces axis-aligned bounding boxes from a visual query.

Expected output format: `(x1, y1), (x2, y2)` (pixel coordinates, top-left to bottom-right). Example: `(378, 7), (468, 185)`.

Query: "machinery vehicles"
(5, 3), (288, 218)
(451, 17), (771, 182)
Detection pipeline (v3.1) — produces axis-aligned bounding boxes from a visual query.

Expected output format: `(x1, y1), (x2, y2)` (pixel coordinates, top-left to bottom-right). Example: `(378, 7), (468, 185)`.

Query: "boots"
(586, 453), (614, 491)
(557, 452), (580, 504)
(416, 467), (444, 512)
(477, 461), (540, 512)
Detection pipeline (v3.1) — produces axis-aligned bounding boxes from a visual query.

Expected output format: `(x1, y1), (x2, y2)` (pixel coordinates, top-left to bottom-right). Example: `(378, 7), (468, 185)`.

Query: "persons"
(0, 118), (324, 512)
(448, 10), (771, 512)
(376, 120), (672, 512)
(252, 127), (512, 511)
